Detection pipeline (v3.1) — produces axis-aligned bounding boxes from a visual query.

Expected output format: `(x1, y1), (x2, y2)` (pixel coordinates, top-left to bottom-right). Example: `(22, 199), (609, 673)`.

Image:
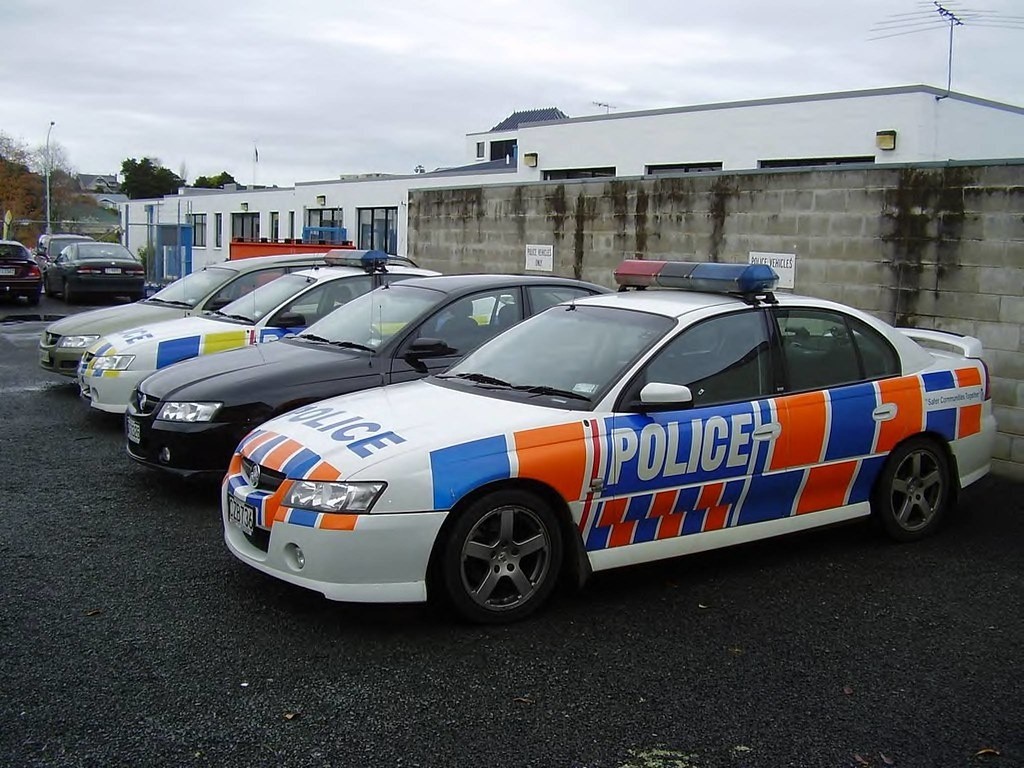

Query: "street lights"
(45, 121), (55, 234)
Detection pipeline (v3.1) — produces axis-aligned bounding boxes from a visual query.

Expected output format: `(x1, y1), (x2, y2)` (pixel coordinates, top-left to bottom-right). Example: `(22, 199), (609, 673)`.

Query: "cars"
(74, 248), (444, 429)
(37, 253), (330, 381)
(43, 242), (145, 304)
(219, 258), (997, 624)
(0, 240), (43, 305)
(122, 272), (616, 491)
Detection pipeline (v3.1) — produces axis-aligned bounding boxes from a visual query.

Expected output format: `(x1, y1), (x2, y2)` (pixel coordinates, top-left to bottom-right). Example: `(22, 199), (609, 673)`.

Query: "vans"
(38, 234), (95, 264)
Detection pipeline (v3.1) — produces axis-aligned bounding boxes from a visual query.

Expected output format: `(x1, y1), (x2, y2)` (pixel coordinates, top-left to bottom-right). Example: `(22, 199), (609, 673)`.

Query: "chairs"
(438, 301), (478, 335)
(476, 304), (519, 339)
(332, 286), (353, 312)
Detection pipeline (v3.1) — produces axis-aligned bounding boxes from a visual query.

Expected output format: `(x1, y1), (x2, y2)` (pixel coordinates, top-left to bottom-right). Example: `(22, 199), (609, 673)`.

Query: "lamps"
(523, 153), (538, 167)
(876, 130), (896, 151)
(316, 195), (326, 206)
(240, 203), (248, 211)
